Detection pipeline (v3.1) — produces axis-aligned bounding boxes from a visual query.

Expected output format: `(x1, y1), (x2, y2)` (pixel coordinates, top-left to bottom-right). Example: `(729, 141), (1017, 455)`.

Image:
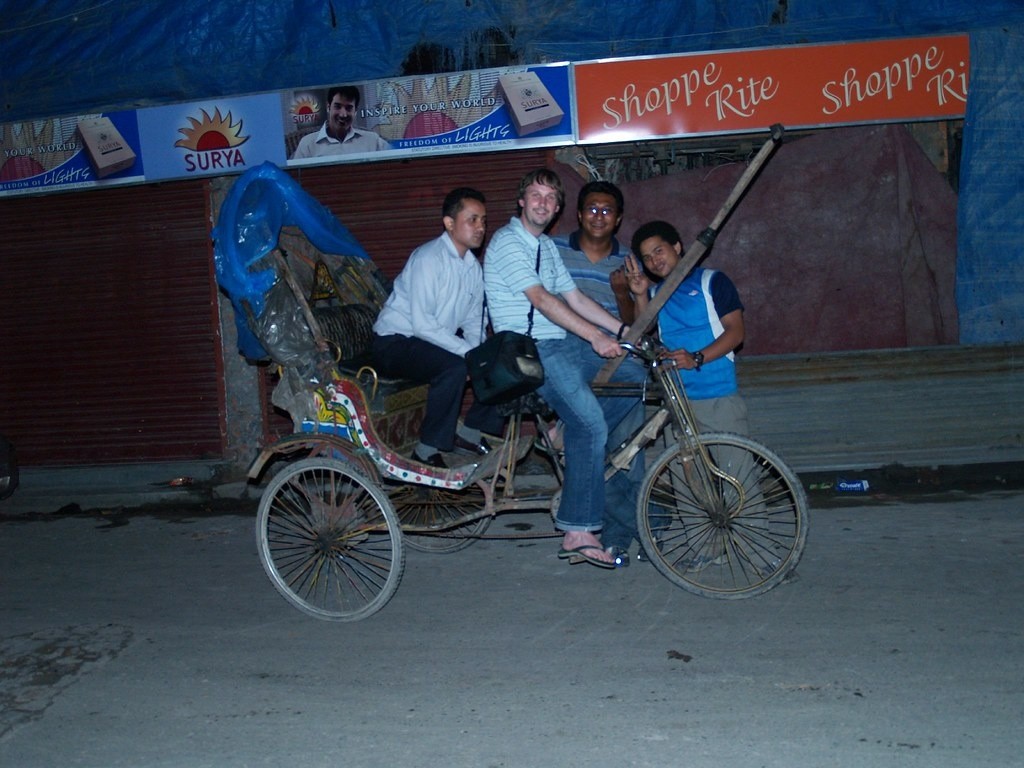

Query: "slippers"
(557, 545), (616, 568)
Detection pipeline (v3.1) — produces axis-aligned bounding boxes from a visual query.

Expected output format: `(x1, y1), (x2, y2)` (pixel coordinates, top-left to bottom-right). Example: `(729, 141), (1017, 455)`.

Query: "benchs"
(308, 303), (433, 413)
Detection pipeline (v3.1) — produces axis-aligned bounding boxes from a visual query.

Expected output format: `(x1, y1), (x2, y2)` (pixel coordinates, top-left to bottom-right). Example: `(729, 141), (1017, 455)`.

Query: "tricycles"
(211, 161), (811, 622)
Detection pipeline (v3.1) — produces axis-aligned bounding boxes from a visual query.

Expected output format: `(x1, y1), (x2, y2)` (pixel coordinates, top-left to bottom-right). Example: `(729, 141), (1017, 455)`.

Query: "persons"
(623, 219), (801, 585)
(482, 168), (645, 569)
(292, 87), (392, 159)
(368, 187), (509, 468)
(551, 179), (672, 568)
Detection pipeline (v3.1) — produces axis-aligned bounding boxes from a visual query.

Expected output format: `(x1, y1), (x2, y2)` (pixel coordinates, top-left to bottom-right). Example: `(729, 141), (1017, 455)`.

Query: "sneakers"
(749, 559), (798, 585)
(674, 550), (730, 573)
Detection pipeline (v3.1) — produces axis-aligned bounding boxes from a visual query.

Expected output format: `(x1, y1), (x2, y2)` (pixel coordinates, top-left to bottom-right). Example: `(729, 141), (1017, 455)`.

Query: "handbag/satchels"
(464, 330), (545, 414)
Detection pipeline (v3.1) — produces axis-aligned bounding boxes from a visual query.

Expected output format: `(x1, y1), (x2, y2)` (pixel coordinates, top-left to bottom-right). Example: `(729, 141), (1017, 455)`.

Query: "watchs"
(693, 351), (705, 372)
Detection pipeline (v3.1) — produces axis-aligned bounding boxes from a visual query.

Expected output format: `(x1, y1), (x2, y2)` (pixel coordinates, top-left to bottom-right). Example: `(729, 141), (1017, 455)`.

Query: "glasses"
(584, 207), (618, 217)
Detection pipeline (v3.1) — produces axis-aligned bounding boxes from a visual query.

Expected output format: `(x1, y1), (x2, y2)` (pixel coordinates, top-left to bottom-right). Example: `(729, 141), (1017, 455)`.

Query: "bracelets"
(617, 322), (627, 341)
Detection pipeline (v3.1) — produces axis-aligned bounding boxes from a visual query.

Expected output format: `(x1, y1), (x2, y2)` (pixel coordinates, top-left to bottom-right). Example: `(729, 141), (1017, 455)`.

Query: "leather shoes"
(455, 432), (492, 459)
(410, 450), (448, 469)
